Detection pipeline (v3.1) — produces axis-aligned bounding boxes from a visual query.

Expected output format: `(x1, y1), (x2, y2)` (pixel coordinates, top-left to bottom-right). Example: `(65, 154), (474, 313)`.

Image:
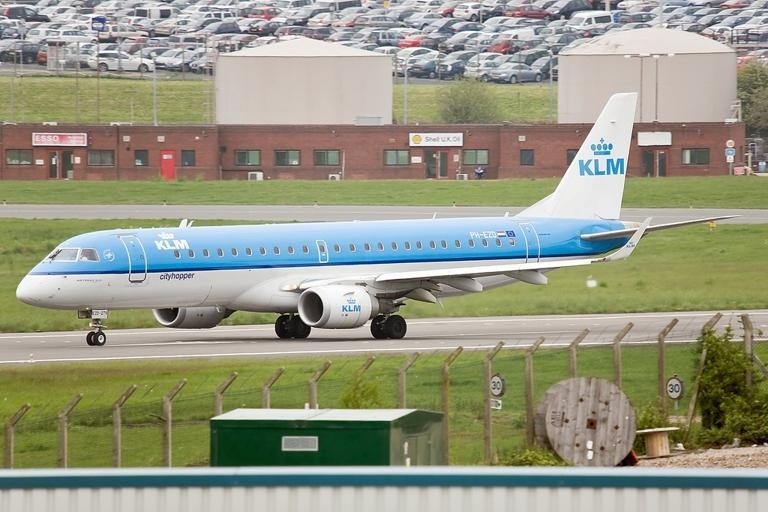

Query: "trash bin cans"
(474, 169), (483, 179)
(758, 162), (766, 173)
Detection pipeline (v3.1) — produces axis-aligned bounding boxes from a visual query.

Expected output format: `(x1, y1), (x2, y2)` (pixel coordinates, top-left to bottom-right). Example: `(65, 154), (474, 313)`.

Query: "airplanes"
(14, 90), (743, 345)
